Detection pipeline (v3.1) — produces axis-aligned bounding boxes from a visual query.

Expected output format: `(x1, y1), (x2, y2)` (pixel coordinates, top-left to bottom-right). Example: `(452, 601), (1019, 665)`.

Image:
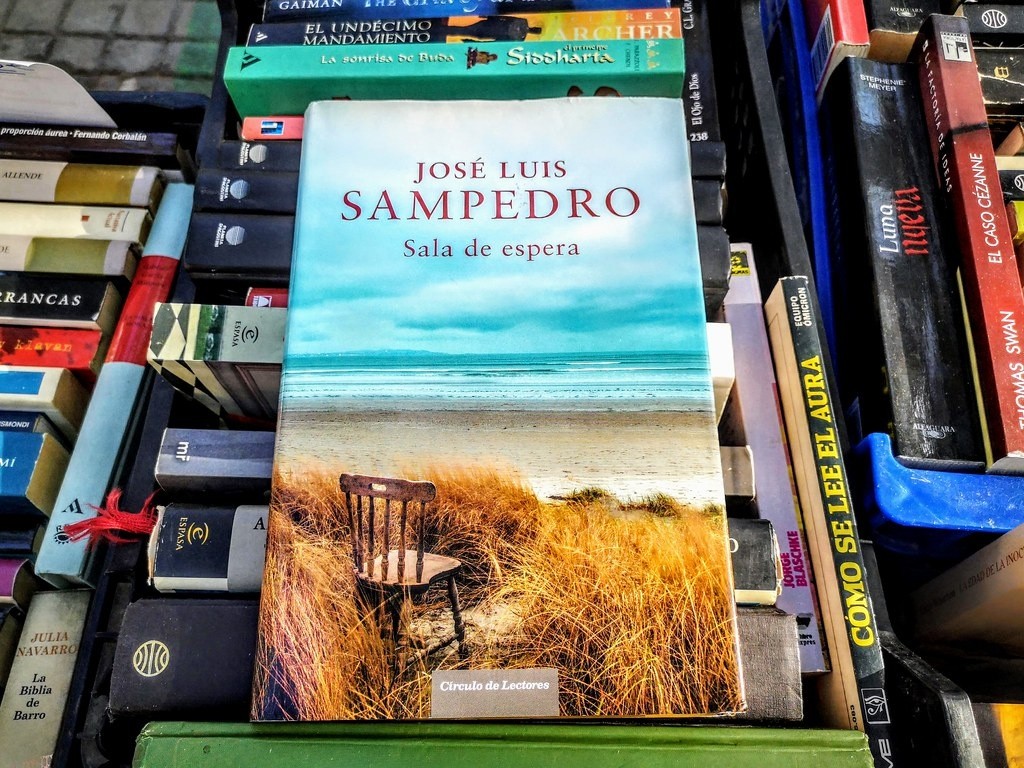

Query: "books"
(861, 523), (1024, 768)
(248, 97), (749, 723)
(1, 125), (195, 768)
(111, 0), (918, 768)
(777, 0), (1024, 481)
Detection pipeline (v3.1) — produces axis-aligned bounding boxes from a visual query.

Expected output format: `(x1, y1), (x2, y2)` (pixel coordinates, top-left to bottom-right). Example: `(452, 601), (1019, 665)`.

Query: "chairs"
(337, 473), (467, 680)
